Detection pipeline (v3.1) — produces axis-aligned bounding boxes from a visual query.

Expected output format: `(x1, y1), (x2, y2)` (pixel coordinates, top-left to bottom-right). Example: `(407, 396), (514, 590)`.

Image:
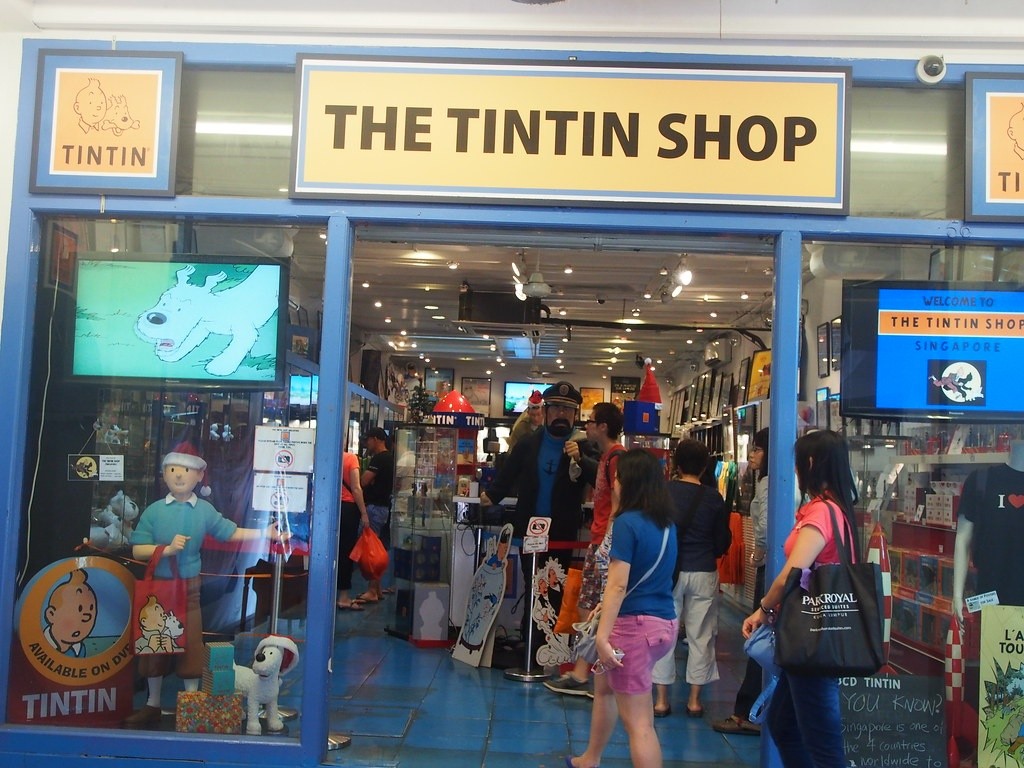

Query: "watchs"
(751, 552), (764, 562)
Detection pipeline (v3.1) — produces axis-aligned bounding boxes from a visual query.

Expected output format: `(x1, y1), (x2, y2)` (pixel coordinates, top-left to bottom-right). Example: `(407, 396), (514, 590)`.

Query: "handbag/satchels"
(742, 620), (783, 725)
(572, 610), (601, 664)
(349, 525), (389, 583)
(775, 501), (887, 678)
(554, 567), (583, 635)
(128, 544), (190, 656)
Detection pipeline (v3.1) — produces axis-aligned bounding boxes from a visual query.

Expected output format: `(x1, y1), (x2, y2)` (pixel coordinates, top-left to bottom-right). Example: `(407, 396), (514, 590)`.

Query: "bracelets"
(759, 597), (774, 614)
(261, 528), (264, 539)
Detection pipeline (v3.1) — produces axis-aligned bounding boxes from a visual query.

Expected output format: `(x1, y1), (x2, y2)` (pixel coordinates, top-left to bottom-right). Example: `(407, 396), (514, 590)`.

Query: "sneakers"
(542, 672), (595, 700)
(713, 714), (761, 736)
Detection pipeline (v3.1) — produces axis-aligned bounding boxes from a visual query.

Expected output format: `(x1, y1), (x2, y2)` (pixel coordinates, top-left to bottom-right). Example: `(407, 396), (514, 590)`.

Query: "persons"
(712, 427), (889, 768)
(338, 427), (394, 611)
(123, 441), (294, 724)
(480, 381), (603, 667)
(544, 402), (725, 768)
(949, 438), (1024, 636)
(507, 390), (545, 454)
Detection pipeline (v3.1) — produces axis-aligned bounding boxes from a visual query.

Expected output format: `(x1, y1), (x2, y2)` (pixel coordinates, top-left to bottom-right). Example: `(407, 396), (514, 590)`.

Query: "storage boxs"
(201, 641), (235, 696)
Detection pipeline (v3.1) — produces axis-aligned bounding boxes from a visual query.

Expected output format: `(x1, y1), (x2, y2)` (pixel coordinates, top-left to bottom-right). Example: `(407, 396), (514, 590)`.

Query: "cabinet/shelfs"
(889, 450), (1010, 677)
(688, 420), (724, 463)
(384, 422), (457, 648)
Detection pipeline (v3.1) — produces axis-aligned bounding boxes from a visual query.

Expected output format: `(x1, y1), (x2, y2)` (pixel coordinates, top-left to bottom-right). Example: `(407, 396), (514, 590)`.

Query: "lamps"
(669, 256), (693, 298)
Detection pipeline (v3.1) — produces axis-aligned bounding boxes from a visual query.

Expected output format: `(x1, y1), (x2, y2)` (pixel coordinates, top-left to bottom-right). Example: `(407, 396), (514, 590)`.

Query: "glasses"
(752, 446), (763, 452)
(587, 419), (607, 424)
(590, 647), (625, 674)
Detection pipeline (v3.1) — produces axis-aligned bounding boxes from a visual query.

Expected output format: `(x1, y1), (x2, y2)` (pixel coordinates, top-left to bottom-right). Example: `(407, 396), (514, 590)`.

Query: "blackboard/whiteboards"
(838, 675), (946, 768)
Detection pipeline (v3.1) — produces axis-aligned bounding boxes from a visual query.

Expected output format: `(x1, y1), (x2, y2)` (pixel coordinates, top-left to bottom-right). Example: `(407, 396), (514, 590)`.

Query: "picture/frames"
(667, 315), (900, 437)
(461, 376), (492, 418)
(424, 367), (455, 401)
(735, 404), (756, 516)
(579, 387), (604, 422)
(287, 299), (323, 364)
(963, 70), (1024, 224)
(28, 48), (185, 198)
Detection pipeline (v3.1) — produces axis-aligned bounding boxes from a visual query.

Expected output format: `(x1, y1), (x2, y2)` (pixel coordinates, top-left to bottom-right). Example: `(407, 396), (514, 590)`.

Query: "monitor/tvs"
(503, 381), (557, 417)
(737, 404), (756, 434)
(61, 251), (292, 392)
(839, 280), (1024, 422)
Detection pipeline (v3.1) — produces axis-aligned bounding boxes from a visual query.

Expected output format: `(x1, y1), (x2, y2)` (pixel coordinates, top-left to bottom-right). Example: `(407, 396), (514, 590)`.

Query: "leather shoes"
(124, 704), (162, 725)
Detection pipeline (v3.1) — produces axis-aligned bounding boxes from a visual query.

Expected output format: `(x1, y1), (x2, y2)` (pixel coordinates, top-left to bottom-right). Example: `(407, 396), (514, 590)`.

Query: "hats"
(527, 390), (543, 408)
(255, 634), (300, 677)
(162, 440), (212, 496)
(634, 357), (663, 412)
(360, 427), (388, 441)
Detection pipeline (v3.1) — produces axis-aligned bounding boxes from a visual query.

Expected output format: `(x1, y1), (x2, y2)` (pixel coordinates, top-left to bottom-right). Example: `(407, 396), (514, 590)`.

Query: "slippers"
(383, 588), (396, 594)
(356, 593), (386, 604)
(653, 702), (703, 718)
(338, 601), (366, 610)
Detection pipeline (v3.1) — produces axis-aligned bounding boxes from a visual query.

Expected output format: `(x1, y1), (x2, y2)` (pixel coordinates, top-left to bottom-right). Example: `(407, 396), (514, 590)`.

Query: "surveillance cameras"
(595, 295), (607, 305)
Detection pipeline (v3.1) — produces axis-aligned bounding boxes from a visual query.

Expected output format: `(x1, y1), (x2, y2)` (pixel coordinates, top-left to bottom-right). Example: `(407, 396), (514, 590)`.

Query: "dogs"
(233, 646), (284, 734)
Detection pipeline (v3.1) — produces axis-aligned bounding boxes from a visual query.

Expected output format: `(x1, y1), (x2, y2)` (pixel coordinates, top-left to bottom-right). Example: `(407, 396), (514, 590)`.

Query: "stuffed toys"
(233, 634), (300, 730)
(90, 490), (139, 547)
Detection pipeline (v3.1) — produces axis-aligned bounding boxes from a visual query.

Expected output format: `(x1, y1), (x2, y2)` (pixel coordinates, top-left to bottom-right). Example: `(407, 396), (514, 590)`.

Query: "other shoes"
(564, 753), (598, 768)
